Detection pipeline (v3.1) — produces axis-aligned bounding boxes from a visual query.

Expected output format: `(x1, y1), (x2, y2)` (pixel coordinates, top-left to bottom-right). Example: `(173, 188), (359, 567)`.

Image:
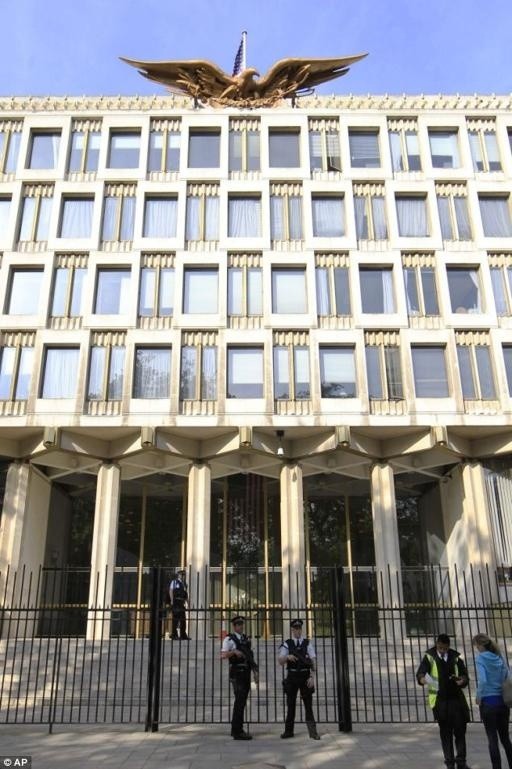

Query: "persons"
(471, 633), (512, 769)
(277, 618), (320, 739)
(220, 616), (260, 740)
(415, 633), (472, 768)
(169, 569), (192, 640)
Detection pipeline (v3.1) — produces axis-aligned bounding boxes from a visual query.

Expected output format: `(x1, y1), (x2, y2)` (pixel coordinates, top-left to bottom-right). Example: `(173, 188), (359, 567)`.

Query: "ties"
(296, 639), (300, 646)
(441, 652), (445, 663)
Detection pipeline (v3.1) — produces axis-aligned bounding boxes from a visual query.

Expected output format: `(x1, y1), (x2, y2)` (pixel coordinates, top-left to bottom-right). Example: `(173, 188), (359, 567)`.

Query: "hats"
(177, 570), (186, 575)
(290, 620), (304, 628)
(230, 617), (244, 625)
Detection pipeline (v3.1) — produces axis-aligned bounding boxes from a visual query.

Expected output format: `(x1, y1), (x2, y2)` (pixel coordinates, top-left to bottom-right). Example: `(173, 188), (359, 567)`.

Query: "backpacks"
(502, 676), (512, 705)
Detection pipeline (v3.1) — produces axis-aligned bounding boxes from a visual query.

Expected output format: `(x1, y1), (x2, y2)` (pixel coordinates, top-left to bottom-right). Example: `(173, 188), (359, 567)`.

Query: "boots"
(444, 759), (471, 769)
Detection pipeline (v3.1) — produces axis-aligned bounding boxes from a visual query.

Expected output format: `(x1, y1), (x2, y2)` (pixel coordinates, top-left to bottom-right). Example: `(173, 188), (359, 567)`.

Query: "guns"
(235, 642), (258, 673)
(288, 651), (318, 673)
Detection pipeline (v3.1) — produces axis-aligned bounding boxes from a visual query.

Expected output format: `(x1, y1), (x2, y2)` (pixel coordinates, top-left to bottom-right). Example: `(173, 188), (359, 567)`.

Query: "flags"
(232, 40), (244, 76)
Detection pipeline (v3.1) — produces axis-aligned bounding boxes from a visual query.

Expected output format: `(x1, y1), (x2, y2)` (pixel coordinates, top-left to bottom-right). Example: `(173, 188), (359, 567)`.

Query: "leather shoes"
(172, 633), (192, 640)
(231, 730), (253, 742)
(309, 730), (321, 740)
(280, 730), (294, 738)
